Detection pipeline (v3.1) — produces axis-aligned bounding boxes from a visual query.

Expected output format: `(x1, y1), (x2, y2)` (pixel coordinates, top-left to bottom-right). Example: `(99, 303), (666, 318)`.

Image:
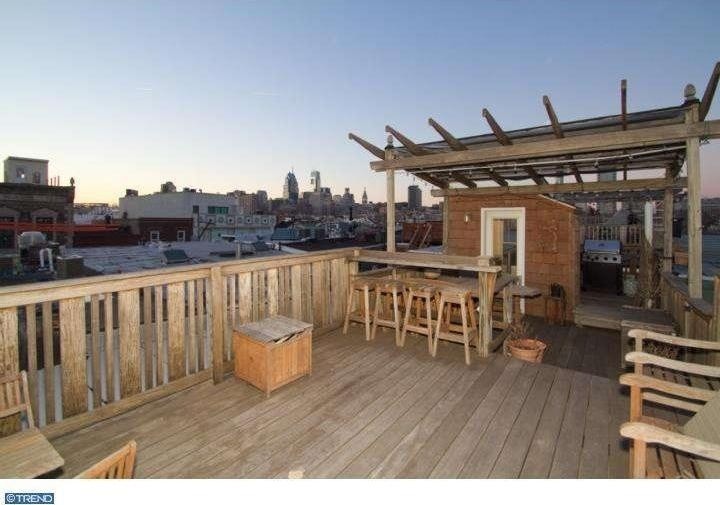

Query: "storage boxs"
(232, 315), (314, 399)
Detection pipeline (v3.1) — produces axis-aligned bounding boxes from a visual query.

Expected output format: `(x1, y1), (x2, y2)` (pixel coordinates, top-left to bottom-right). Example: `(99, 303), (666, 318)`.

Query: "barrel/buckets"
(220, 234), (236, 257)
(0, 256), (14, 286)
(240, 241), (254, 254)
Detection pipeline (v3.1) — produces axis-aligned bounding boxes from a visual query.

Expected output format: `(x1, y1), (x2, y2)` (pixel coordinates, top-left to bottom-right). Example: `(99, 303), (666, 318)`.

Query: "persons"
(105, 214), (113, 224)
(626, 208), (639, 242)
(588, 204), (593, 215)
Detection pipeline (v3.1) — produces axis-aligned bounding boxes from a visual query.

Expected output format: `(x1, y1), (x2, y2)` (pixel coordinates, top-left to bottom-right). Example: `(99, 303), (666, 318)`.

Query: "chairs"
(0, 369), (138, 478)
(618, 329), (720, 478)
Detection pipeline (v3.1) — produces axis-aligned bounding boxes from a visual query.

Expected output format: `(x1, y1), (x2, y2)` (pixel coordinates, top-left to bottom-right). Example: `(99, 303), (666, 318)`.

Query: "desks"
(346, 250), (501, 356)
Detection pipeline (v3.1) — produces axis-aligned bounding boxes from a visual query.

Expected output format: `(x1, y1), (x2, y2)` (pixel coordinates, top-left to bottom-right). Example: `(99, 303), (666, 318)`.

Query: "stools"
(342, 277), (478, 365)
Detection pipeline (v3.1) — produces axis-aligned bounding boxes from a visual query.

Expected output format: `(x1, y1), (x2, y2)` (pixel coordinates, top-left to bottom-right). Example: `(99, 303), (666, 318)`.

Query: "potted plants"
(506, 315), (548, 365)
